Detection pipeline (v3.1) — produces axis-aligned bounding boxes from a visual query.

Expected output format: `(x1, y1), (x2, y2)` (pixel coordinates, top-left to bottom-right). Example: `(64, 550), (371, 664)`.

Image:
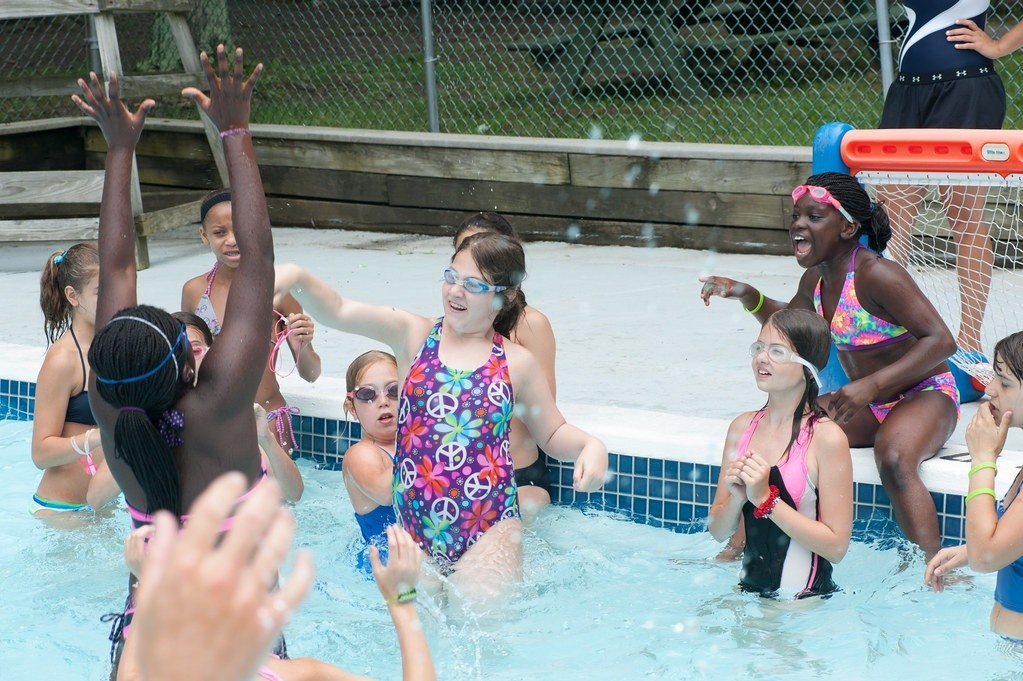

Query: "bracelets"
(386, 587), (418, 603)
(743, 290), (764, 314)
(965, 488), (996, 503)
(71, 429), (97, 474)
(968, 462), (997, 477)
(219, 127), (253, 139)
(753, 485), (780, 519)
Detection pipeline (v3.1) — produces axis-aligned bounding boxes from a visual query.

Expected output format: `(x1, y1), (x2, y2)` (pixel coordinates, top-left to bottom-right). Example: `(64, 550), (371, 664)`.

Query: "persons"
(923, 331), (1023, 639)
(428, 211), (556, 504)
(697, 172), (961, 548)
(270, 232), (609, 561)
(875, 0), (1023, 353)
(342, 350), (399, 540)
(86, 310), (304, 510)
(69, 45), (289, 681)
(180, 187), (322, 455)
(31, 241), (118, 510)
(116, 472), (437, 681)
(708, 308), (853, 596)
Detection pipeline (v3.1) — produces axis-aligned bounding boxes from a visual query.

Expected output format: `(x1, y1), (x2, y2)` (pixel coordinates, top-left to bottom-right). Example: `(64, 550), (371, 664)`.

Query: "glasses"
(192, 344), (211, 361)
(441, 263), (509, 295)
(791, 184), (854, 224)
(346, 381), (398, 402)
(746, 340), (823, 389)
